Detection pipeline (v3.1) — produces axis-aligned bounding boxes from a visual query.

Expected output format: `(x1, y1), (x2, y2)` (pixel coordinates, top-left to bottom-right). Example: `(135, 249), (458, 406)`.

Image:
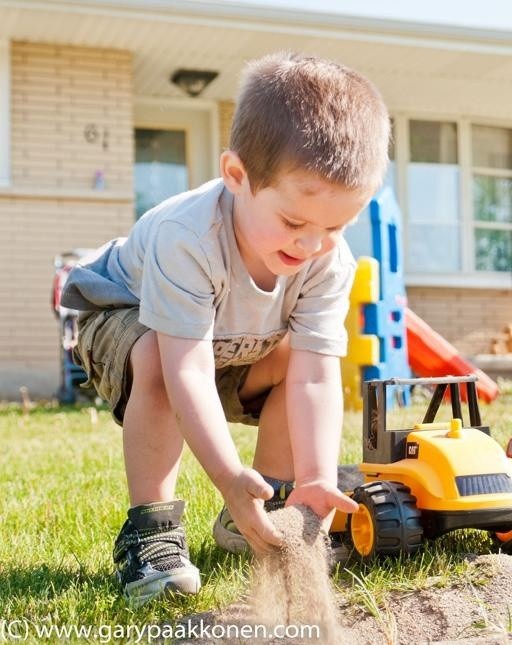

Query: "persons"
(59, 49), (390, 610)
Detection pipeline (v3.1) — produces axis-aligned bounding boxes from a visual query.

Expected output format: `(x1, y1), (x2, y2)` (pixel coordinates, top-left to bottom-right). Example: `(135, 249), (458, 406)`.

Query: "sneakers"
(213, 473), (349, 571)
(111, 499), (200, 609)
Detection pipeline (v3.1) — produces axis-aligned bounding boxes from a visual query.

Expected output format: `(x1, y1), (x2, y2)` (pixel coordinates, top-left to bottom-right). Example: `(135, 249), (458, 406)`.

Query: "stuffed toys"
(328, 374), (512, 559)
